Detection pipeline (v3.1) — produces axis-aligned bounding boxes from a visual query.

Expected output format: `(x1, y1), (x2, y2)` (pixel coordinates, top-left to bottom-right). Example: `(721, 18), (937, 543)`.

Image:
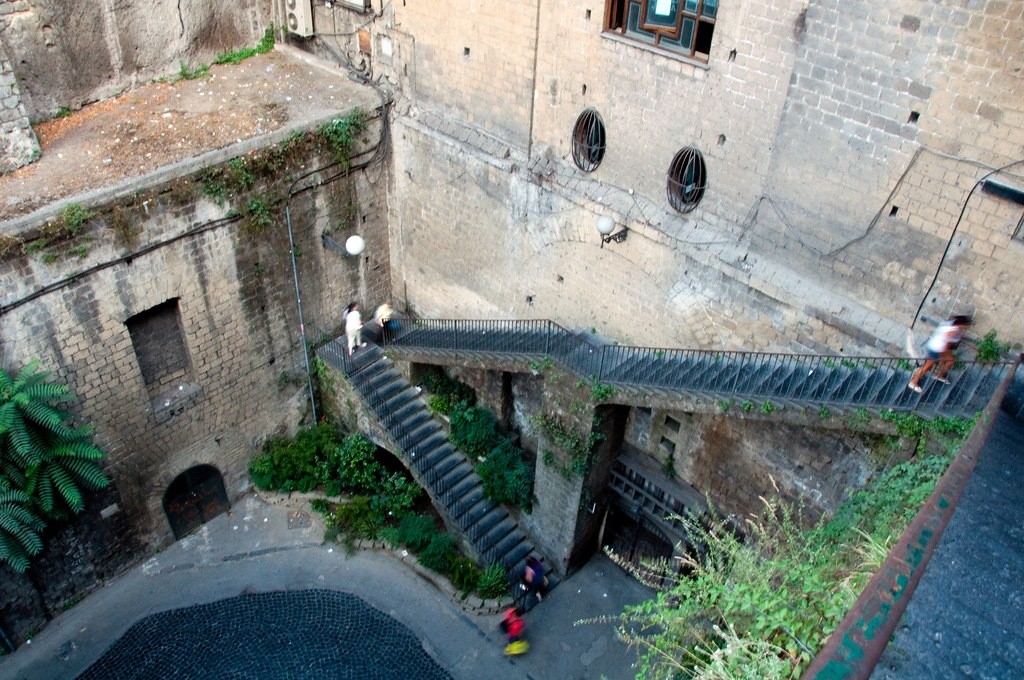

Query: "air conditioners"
(284, 0), (313, 37)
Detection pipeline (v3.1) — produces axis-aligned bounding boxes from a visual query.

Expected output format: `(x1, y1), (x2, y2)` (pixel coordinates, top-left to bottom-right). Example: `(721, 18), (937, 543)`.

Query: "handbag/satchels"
(502, 637), (530, 655)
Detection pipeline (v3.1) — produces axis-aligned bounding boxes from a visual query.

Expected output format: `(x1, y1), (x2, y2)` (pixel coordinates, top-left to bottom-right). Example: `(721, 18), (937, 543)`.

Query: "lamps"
(346, 235), (365, 255)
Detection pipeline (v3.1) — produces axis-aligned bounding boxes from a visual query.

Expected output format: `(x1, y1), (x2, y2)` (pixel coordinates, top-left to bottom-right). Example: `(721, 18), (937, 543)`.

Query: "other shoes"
(933, 375), (951, 385)
(909, 381), (922, 394)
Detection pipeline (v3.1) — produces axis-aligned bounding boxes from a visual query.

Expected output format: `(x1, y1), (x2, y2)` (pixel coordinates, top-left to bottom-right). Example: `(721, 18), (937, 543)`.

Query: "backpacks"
(499, 615), (522, 635)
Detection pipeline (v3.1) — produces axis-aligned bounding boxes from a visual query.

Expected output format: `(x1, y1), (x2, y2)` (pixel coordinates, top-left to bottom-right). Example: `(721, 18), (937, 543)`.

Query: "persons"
(498, 605), (529, 647)
(373, 298), (397, 343)
(343, 302), (368, 357)
(519, 555), (546, 603)
(907, 315), (973, 393)
